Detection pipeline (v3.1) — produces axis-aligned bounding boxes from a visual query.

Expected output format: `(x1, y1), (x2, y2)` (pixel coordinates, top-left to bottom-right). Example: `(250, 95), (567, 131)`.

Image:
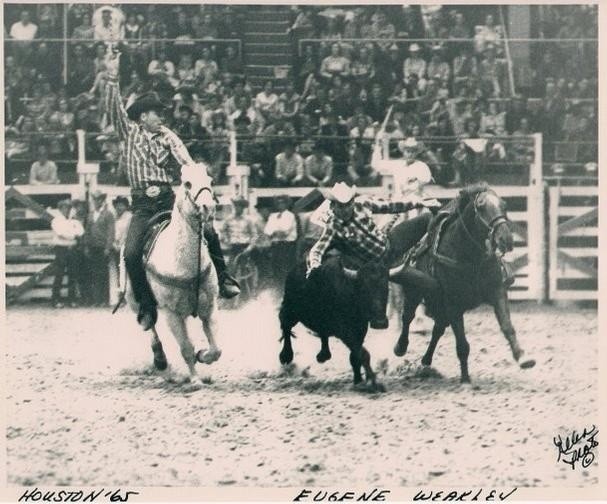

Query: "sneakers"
(221, 280), (240, 298)
(142, 305), (153, 331)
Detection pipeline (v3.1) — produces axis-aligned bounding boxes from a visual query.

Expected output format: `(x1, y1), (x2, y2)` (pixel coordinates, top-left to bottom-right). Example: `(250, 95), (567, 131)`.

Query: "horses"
(390, 180), (536, 390)
(119, 161), (223, 386)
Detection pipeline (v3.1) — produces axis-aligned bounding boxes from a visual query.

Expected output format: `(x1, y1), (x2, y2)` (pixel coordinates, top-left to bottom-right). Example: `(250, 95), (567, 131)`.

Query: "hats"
(127, 92), (167, 120)
(58, 189), (129, 209)
(175, 74), (390, 147)
(60, 6), (122, 52)
(397, 136), (425, 152)
(231, 192), (292, 208)
(328, 182), (358, 202)
(396, 43), (509, 136)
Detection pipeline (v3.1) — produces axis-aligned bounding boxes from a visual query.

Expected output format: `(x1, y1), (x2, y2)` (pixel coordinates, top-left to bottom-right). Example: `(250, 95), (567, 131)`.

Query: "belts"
(131, 185), (172, 198)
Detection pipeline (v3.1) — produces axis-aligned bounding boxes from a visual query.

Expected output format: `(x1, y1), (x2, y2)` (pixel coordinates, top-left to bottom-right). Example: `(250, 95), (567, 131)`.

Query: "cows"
(278, 254), (406, 389)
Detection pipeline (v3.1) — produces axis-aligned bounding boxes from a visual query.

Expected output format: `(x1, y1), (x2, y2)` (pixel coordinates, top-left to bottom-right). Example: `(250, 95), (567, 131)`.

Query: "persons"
(97, 40), (241, 333)
(304, 181), (442, 319)
